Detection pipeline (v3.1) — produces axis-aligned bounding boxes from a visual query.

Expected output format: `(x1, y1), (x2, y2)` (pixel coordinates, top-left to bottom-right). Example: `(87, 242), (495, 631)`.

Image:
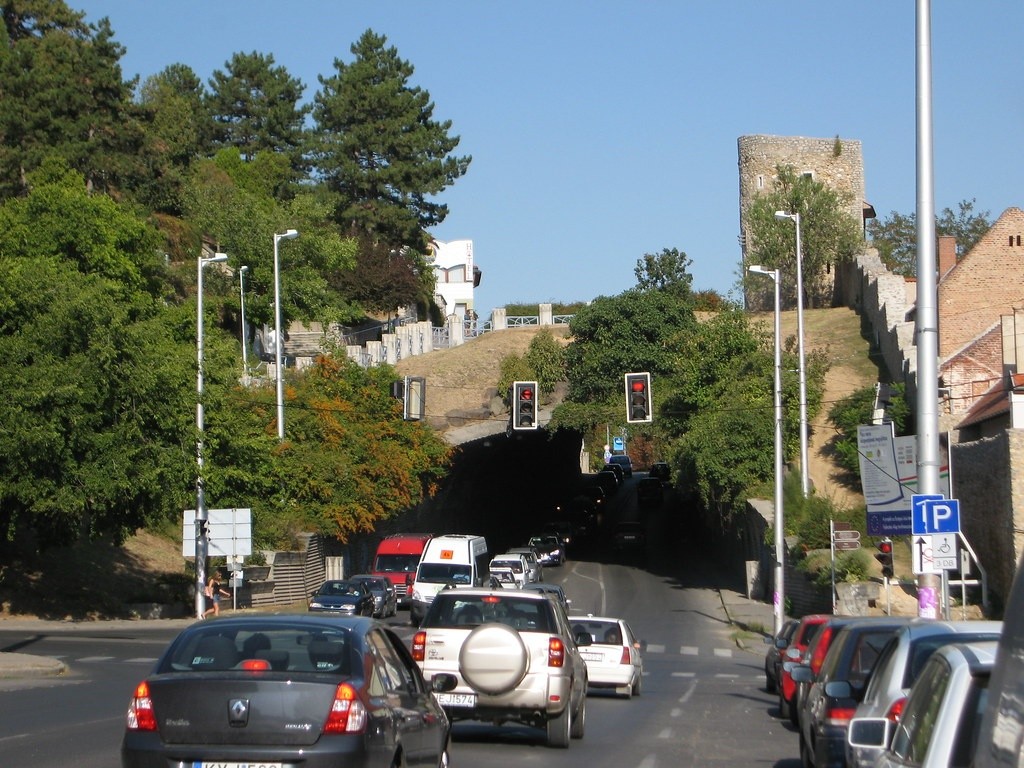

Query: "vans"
(372, 532), (488, 627)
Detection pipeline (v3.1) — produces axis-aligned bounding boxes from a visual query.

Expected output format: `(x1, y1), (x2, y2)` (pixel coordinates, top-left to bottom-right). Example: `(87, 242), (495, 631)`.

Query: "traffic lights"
(625, 372), (652, 423)
(512, 381), (537, 430)
(873, 541), (893, 578)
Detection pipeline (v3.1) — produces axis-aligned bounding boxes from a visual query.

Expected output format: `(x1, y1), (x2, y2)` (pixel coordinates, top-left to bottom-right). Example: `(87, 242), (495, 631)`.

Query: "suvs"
(411, 582), (589, 749)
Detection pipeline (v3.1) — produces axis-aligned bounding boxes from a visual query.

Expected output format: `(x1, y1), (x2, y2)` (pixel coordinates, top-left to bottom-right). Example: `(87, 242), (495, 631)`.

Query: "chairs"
(456, 605), (483, 627)
(258, 651), (288, 673)
(194, 635), (237, 673)
(307, 642), (344, 668)
(243, 633), (272, 663)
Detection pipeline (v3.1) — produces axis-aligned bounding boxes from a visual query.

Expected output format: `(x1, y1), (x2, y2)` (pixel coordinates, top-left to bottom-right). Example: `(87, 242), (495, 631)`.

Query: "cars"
(763, 620), (799, 694)
(611, 464), (672, 552)
(121, 614), (458, 768)
(849, 642), (1000, 768)
(566, 614), (644, 699)
(824, 620), (1003, 768)
(790, 617), (918, 768)
(307, 574), (398, 621)
(774, 615), (849, 720)
(783, 616), (873, 728)
(487, 455), (632, 617)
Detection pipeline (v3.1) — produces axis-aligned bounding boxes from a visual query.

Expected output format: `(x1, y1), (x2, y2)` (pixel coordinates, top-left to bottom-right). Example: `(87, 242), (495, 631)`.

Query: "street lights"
(195, 253), (228, 627)
(748, 266), (784, 636)
(775, 210), (808, 498)
(239, 229), (298, 439)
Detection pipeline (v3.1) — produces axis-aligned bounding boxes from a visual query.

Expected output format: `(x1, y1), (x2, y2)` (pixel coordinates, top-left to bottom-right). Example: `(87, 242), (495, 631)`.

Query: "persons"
(347, 584), (357, 593)
(201, 570), (231, 619)
